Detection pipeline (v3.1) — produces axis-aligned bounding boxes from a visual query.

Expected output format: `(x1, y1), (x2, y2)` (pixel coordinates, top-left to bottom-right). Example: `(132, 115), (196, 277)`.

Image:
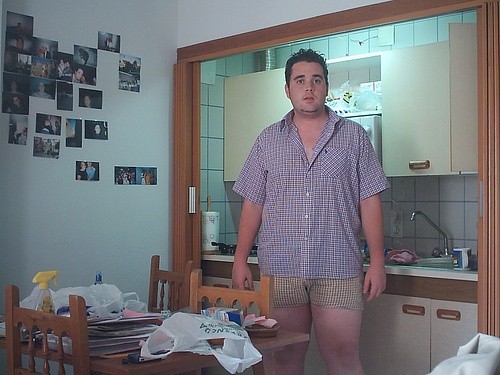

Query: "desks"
(1, 331), (311, 375)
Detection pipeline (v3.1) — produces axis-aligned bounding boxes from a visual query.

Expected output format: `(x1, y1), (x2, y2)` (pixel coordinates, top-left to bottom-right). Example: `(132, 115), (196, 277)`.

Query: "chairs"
(147, 255), (195, 312)
(5, 285), (93, 375)
(188, 269), (275, 324)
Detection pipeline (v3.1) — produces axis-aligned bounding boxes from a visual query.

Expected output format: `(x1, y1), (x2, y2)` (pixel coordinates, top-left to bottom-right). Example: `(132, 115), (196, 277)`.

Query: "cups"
(453, 247), (472, 271)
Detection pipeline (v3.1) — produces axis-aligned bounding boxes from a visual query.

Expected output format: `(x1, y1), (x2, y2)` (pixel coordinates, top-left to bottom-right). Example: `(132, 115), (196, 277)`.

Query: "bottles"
(94, 271), (103, 285)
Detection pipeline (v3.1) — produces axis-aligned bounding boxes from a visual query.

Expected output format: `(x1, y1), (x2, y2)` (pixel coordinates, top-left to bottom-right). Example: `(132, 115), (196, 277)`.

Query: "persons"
(232, 49), (391, 375)
(3, 22), (158, 185)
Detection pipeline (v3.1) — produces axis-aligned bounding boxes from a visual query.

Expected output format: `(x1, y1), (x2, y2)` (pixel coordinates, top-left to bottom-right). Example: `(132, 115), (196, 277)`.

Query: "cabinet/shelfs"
(381, 23), (479, 180)
(207, 277), (478, 375)
(223, 70), (294, 182)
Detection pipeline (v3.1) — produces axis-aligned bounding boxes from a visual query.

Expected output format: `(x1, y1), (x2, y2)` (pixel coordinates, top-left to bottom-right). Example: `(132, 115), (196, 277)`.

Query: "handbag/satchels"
(140, 312), (262, 373)
(20, 284), (123, 317)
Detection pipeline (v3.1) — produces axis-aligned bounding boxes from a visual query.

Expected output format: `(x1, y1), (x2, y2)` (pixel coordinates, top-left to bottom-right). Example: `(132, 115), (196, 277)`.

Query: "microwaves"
(346, 116), (382, 168)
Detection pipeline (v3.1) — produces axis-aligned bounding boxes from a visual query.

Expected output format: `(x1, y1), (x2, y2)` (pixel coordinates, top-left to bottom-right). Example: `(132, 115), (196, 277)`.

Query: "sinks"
(400, 258), (477, 269)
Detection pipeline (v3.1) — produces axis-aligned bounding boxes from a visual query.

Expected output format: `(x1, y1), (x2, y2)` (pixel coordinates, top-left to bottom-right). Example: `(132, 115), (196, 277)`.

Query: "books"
(0, 308), (173, 356)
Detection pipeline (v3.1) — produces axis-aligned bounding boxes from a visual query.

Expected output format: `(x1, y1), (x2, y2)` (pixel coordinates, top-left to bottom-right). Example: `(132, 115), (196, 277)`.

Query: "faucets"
(410, 210), (453, 258)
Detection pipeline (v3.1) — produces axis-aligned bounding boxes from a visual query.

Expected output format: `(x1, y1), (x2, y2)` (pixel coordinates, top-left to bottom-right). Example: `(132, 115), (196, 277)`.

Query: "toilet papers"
(201, 211), (220, 251)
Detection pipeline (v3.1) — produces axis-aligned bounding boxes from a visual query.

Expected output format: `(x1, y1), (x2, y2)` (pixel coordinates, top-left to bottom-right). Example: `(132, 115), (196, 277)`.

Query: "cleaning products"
(31, 270), (58, 315)
